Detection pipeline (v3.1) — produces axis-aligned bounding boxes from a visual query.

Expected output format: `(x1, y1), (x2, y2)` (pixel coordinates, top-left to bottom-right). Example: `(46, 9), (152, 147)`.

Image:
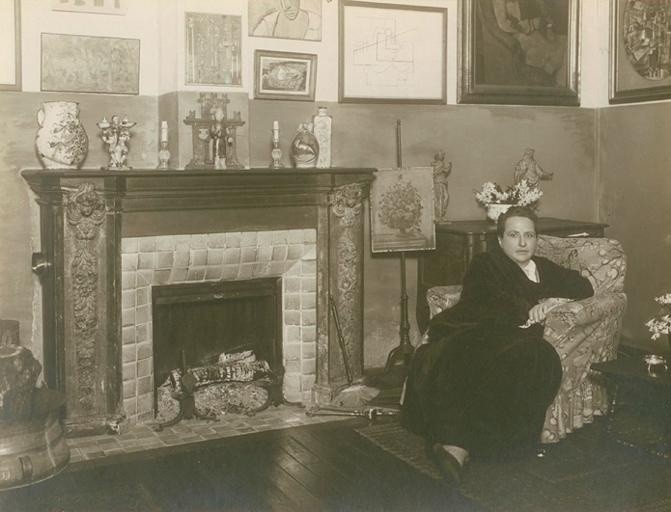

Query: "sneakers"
(432, 441), (463, 487)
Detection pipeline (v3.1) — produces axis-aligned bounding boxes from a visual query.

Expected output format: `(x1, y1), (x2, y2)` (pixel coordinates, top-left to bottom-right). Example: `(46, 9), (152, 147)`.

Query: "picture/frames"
(336, 0), (447, 106)
(49, 0), (130, 16)
(250, 49), (320, 102)
(456, 1), (582, 106)
(40, 31), (140, 94)
(369, 166), (437, 254)
(608, 1), (670, 104)
(0, 0), (24, 90)
(184, 11), (243, 88)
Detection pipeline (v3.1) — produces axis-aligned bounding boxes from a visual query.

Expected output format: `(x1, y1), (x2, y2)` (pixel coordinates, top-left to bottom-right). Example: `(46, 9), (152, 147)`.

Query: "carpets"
(354, 405), (669, 510)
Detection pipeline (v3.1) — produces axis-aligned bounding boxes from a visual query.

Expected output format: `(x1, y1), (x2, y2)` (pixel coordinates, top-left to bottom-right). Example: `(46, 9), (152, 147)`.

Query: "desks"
(415, 216), (613, 337)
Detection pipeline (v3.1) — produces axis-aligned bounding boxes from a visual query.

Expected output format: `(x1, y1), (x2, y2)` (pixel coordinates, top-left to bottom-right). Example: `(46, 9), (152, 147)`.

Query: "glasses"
(507, 230), (535, 240)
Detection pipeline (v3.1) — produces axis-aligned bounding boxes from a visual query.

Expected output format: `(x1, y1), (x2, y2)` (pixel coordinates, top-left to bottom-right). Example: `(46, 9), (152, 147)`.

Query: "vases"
(34, 100), (90, 169)
(487, 197), (515, 219)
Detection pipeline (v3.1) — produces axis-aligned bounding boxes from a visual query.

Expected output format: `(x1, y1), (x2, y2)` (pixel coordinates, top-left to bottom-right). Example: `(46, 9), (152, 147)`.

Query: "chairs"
(409, 235), (626, 446)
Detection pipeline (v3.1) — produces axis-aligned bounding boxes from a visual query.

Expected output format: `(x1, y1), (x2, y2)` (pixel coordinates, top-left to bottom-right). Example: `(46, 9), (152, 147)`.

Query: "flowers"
(473, 182), (541, 208)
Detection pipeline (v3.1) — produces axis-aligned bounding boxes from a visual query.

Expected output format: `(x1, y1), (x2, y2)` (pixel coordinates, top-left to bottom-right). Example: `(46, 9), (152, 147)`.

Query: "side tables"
(590, 354), (670, 457)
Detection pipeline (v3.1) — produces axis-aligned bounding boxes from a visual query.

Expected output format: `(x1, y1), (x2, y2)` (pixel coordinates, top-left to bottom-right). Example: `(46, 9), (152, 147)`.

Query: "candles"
(273, 121), (280, 141)
(162, 120), (168, 140)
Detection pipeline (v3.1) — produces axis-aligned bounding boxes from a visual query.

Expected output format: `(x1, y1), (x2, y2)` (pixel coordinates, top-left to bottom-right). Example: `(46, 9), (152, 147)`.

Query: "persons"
(492, 1), (568, 91)
(513, 146), (553, 188)
(209, 108), (229, 170)
(399, 206), (597, 485)
(431, 151), (454, 226)
